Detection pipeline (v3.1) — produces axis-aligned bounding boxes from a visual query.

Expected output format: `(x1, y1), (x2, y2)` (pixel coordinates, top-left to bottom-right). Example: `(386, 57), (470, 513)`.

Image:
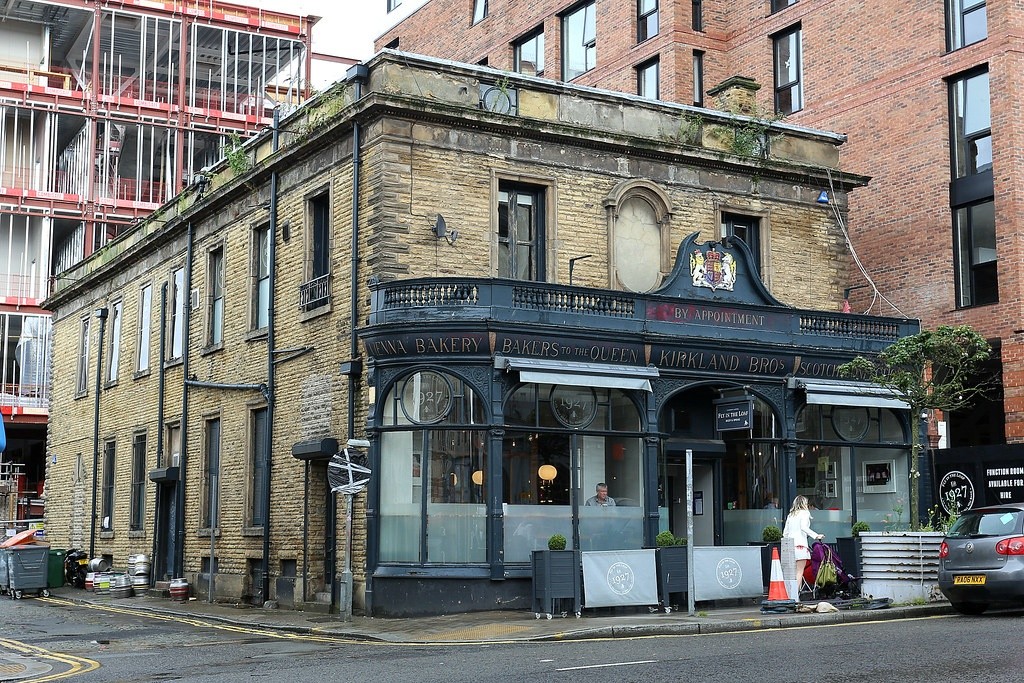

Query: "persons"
(764, 496), (779, 509)
(783, 495), (825, 593)
(584, 483), (616, 506)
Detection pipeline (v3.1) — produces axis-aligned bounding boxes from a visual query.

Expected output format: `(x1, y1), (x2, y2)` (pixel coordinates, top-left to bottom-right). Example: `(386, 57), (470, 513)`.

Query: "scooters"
(57, 547), (91, 590)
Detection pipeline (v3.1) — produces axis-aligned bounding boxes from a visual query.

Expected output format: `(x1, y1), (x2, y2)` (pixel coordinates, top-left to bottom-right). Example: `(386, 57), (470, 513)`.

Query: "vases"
(853, 534), (949, 602)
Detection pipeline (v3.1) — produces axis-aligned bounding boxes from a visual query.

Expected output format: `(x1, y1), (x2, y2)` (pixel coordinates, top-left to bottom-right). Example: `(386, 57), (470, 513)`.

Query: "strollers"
(798, 532), (861, 603)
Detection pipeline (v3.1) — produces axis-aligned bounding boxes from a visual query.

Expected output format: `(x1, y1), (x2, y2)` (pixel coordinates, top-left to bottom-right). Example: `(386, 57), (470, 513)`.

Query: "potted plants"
(835, 520), (872, 598)
(531, 534), (583, 620)
(748, 524), (783, 605)
(641, 530), (688, 613)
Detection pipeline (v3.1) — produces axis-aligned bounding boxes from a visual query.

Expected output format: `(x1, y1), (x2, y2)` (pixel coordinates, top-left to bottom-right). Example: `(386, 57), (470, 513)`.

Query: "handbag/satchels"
(816, 549), (838, 586)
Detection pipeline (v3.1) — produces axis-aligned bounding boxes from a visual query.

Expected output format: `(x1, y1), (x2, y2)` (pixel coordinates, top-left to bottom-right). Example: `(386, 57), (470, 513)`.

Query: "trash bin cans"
(46, 549), (66, 588)
(0, 529), (50, 601)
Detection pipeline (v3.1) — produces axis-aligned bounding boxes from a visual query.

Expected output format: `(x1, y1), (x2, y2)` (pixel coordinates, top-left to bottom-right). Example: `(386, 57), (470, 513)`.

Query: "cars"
(936, 502), (1024, 616)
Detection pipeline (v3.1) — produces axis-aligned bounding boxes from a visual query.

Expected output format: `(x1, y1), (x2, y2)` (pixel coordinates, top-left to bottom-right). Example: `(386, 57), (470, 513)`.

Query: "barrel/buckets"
(85, 553), (151, 598)
(170, 578), (189, 601)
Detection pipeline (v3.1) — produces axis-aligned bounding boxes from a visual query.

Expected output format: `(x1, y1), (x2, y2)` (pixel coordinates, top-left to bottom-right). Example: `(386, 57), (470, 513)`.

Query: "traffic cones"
(759, 546), (796, 615)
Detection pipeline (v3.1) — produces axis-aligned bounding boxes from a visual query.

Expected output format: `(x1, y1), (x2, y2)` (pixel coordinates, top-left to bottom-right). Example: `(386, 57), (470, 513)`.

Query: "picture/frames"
(825, 461), (837, 480)
(413, 451), (423, 487)
(861, 458), (897, 495)
(826, 480), (837, 499)
(796, 464), (820, 496)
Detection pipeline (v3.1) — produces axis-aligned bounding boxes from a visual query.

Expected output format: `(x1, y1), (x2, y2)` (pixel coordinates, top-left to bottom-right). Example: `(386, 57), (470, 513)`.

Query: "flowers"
(835, 325), (1003, 532)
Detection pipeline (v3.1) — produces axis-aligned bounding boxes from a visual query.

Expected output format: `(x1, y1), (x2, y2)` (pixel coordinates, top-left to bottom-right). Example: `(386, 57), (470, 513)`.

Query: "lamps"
(538, 465), (558, 480)
(472, 471), (482, 485)
